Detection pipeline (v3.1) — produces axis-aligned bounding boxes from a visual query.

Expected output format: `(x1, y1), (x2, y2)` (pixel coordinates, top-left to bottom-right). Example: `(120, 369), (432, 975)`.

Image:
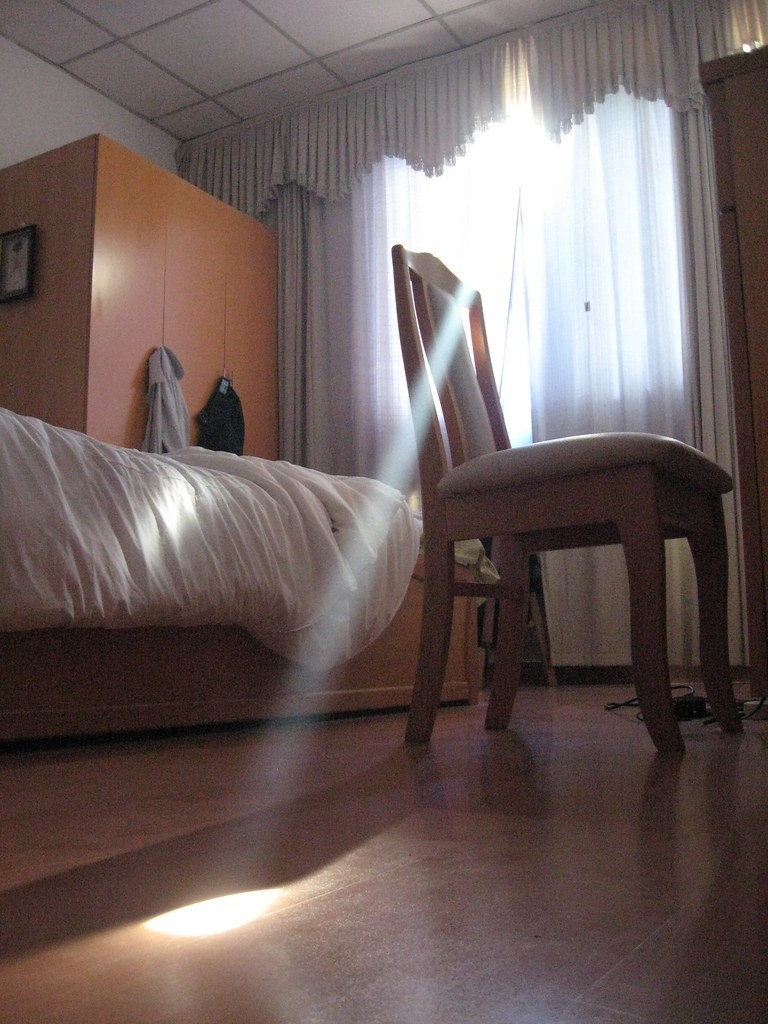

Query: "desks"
(698, 46), (768, 698)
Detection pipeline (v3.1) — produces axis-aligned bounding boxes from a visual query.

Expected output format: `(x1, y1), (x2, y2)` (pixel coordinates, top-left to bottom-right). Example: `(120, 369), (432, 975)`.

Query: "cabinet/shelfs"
(1, 134), (281, 460)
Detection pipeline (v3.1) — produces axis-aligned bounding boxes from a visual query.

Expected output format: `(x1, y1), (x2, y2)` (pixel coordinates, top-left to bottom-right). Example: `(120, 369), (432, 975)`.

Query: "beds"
(0, 405), (496, 743)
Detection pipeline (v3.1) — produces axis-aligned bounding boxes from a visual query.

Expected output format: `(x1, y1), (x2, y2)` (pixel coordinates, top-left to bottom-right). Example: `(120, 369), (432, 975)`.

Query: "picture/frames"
(0, 224), (39, 305)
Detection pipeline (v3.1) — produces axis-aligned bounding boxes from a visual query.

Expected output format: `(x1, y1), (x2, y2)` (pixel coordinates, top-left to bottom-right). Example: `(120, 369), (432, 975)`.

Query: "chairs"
(391, 244), (741, 756)
